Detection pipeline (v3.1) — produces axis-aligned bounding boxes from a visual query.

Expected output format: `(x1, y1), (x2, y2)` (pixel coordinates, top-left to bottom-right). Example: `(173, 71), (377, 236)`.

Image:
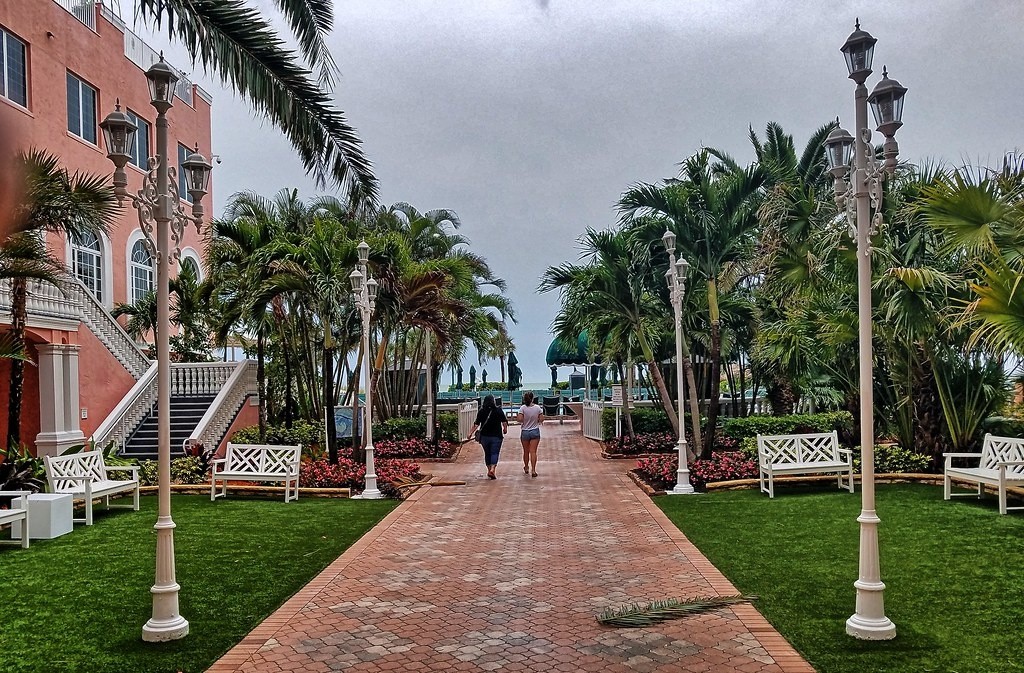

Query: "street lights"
(663, 225), (702, 494)
(97, 48), (214, 642)
(349, 235), (387, 500)
(821, 16), (908, 642)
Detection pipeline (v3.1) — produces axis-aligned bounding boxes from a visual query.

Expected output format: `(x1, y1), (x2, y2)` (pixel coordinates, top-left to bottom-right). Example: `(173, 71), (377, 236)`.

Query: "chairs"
(542, 395), (560, 417)
(562, 395), (579, 416)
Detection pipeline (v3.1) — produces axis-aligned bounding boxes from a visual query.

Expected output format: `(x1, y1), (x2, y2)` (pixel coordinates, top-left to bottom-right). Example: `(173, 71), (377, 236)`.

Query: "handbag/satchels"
(475, 430), (480, 442)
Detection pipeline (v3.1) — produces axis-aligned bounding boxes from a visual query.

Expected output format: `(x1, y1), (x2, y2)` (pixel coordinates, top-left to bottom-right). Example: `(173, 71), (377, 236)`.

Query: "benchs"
(44, 447), (140, 525)
(209, 440), (302, 504)
(942, 432), (1024, 515)
(756, 430), (855, 499)
(0, 490), (33, 549)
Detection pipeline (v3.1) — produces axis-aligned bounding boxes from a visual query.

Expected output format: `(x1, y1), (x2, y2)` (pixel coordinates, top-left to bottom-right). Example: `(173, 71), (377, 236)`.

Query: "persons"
(517, 391), (544, 477)
(467, 393), (508, 480)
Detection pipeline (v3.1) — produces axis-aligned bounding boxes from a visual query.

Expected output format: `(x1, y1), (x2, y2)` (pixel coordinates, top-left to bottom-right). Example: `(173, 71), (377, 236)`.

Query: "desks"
(11, 494), (73, 540)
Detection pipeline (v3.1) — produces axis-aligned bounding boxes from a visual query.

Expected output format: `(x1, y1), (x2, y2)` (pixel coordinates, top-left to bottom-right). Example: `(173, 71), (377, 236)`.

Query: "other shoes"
(487, 471), (497, 479)
(532, 472), (537, 477)
(524, 466), (529, 474)
